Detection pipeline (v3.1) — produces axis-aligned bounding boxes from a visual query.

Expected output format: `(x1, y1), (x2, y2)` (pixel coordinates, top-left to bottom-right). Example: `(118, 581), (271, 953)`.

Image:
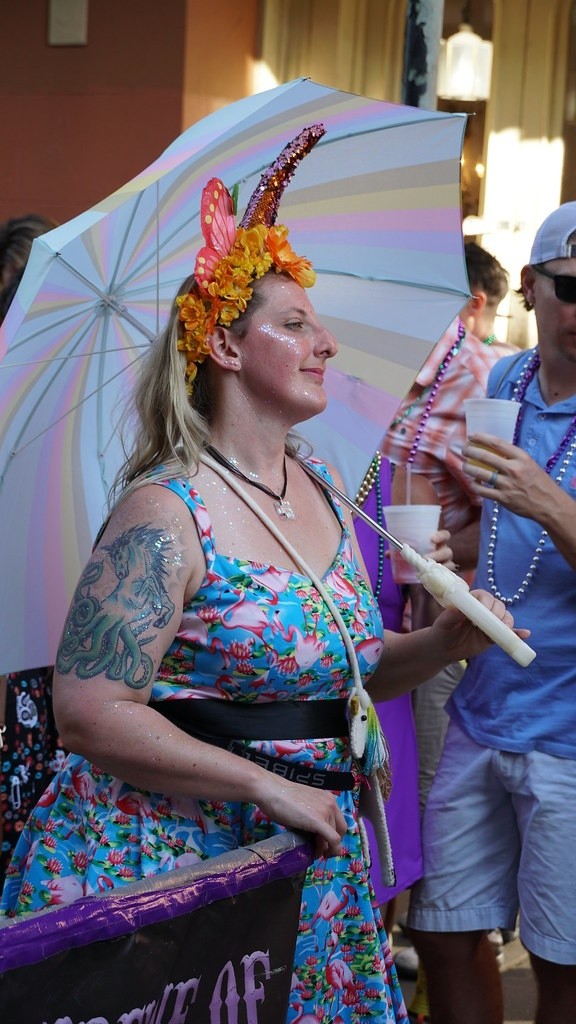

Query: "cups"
(463, 398), (522, 473)
(382, 504), (442, 585)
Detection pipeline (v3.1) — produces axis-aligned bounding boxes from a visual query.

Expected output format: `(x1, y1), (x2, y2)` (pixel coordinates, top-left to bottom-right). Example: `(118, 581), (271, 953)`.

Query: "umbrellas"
(0, 78), (538, 676)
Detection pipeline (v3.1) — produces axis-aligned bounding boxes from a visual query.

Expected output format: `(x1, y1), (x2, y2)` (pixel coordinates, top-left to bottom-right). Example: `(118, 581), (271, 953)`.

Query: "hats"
(529, 200), (576, 265)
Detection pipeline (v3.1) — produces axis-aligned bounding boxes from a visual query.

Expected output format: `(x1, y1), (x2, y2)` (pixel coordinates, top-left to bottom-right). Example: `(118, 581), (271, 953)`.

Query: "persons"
(0, 214), (75, 905)
(348, 450), (460, 945)
(0, 265), (530, 1023)
(406, 198), (576, 1024)
(380, 237), (519, 1024)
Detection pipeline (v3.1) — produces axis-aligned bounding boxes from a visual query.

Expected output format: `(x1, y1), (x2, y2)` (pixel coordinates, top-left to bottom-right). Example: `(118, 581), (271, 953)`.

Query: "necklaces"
(351, 451), (383, 602)
(487, 348), (576, 604)
(391, 322), (466, 460)
(483, 334), (497, 345)
(203, 440), (294, 520)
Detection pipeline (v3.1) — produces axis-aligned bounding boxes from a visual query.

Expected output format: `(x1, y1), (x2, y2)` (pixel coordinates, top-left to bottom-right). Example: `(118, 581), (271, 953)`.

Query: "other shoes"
(393, 928), (503, 976)
(396, 916), (518, 943)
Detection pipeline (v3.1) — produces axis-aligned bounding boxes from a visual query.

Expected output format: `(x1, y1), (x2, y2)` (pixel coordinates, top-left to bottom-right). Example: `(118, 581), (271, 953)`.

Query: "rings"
(489, 472), (496, 483)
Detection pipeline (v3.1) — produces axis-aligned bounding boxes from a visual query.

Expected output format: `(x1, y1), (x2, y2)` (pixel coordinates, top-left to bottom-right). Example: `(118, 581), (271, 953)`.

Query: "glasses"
(533, 265), (576, 305)
